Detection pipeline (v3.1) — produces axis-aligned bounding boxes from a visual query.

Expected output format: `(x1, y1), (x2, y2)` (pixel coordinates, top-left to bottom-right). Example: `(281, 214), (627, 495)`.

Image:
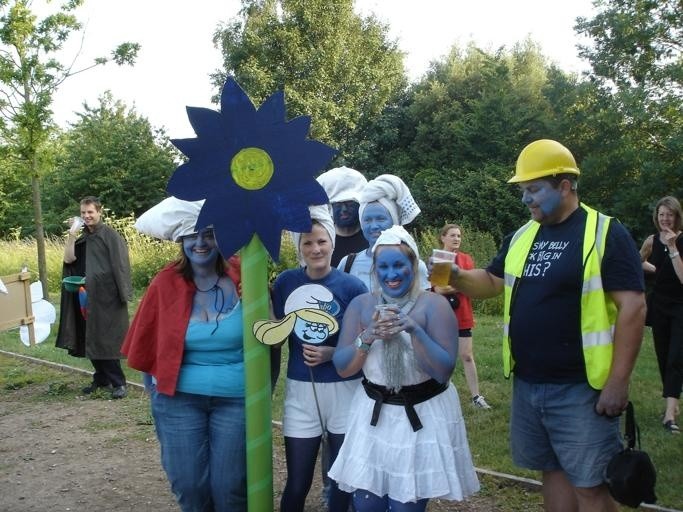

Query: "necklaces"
(194, 271), (235, 335)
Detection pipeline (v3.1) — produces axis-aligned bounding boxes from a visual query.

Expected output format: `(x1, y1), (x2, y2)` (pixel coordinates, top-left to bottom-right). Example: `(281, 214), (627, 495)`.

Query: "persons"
(427, 137), (649, 512)
(327, 224), (483, 511)
(639, 195), (683, 430)
(239, 203), (370, 512)
(439, 224), (491, 413)
(316, 165), (370, 268)
(336, 172), (427, 291)
(120, 227), (247, 512)
(62, 196), (136, 399)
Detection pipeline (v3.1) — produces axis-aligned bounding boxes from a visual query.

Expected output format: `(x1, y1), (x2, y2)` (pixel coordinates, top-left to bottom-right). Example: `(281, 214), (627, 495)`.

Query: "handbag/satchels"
(606, 400), (658, 508)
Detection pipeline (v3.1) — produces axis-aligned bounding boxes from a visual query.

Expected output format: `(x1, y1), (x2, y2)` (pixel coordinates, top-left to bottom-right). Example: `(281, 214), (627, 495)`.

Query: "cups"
(427, 248), (456, 289)
(375, 303), (399, 322)
(66, 215), (84, 238)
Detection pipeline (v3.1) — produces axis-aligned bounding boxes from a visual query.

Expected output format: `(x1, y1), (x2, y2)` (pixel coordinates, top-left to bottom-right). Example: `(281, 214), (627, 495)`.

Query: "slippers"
(661, 405), (681, 435)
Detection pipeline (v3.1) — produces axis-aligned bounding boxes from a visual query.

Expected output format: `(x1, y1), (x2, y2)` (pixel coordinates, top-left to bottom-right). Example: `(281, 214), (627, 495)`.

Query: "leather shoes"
(111, 385), (127, 399)
(81, 382), (113, 395)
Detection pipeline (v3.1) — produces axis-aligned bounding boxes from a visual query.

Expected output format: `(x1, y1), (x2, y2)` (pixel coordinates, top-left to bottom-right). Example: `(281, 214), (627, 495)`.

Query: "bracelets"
(667, 250), (680, 258)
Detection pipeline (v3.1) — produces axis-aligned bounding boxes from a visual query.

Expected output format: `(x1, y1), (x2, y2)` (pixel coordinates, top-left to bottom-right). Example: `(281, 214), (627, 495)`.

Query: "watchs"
(354, 330), (376, 352)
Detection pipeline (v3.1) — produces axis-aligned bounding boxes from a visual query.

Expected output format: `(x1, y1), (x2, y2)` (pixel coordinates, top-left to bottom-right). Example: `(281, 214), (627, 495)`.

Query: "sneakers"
(472, 394), (491, 410)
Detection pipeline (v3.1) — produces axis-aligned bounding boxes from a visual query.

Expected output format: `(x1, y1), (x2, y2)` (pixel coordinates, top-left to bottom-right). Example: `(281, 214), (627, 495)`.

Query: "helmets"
(508, 138), (581, 185)
